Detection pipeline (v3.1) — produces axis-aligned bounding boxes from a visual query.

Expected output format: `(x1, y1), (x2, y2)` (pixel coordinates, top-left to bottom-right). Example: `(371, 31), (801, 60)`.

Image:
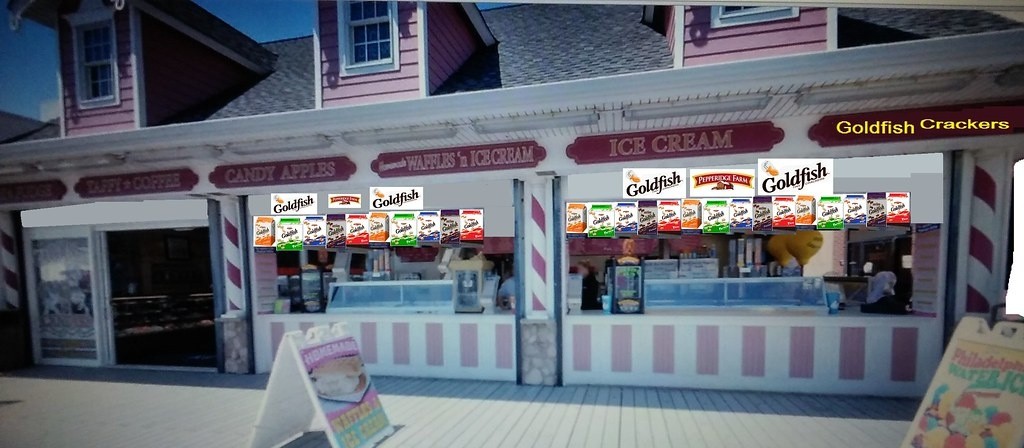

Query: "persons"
(867, 263), (898, 315)
(574, 258), (601, 312)
(496, 262), (516, 312)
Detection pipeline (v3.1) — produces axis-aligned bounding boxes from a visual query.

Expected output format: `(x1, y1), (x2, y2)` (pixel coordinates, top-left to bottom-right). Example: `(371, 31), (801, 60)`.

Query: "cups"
(602, 295), (612, 315)
(826, 293), (840, 315)
